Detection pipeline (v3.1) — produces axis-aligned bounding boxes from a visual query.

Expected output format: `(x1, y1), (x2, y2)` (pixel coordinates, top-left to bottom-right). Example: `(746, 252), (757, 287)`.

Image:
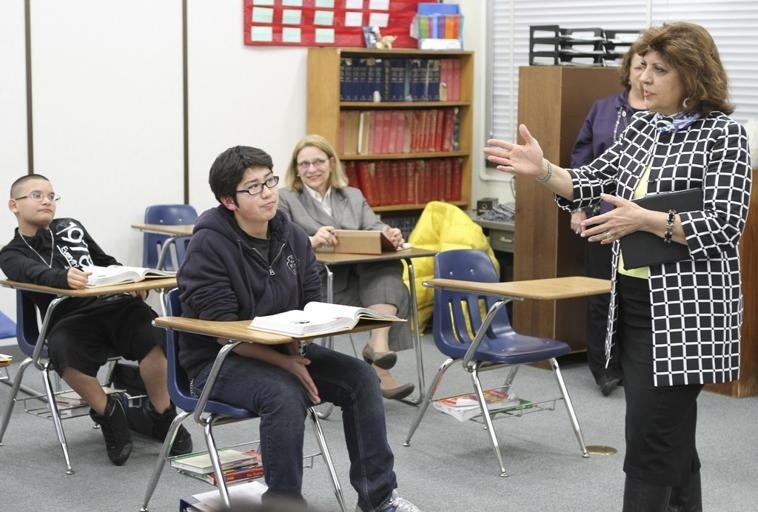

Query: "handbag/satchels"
(111, 363), (176, 437)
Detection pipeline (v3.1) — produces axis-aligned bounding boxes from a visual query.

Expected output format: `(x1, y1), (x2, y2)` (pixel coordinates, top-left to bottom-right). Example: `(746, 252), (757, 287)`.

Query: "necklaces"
(19, 228), (55, 269)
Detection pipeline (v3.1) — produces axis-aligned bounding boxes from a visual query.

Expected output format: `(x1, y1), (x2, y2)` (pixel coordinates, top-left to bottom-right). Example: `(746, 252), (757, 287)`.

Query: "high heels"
(382, 382), (414, 399)
(362, 343), (397, 370)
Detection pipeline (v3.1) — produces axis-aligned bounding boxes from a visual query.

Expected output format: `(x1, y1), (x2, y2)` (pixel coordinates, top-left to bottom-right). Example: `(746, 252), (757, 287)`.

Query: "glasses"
(297, 158), (326, 167)
(15, 190), (61, 202)
(236, 176), (279, 195)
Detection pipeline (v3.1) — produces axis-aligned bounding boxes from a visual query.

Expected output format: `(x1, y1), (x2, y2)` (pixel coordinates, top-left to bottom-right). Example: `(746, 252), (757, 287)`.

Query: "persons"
(567, 43), (648, 394)
(482, 20), (752, 512)
(2, 174), (193, 465)
(277, 134), (415, 400)
(176, 145), (420, 512)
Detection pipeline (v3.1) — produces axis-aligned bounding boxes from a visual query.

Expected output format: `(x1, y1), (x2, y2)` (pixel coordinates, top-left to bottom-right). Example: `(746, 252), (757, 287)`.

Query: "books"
(340, 107), (461, 153)
(382, 215), (419, 241)
(250, 300), (401, 337)
(82, 256), (174, 287)
(344, 156), (463, 206)
(341, 57), (461, 101)
(434, 392), (532, 424)
(168, 447), (264, 485)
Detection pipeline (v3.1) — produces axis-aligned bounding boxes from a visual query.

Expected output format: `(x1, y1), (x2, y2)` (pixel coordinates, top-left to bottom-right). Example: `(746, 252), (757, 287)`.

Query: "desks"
(314, 246), (438, 305)
(426, 276), (612, 371)
(131, 223), (194, 271)
(0, 278), (177, 370)
(150, 316), (394, 428)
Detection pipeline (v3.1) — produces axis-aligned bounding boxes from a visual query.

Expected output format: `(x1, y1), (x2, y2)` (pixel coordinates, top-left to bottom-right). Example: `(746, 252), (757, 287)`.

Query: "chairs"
(142, 205), (197, 274)
(0, 289), (147, 475)
(403, 249), (590, 478)
(139, 289), (347, 511)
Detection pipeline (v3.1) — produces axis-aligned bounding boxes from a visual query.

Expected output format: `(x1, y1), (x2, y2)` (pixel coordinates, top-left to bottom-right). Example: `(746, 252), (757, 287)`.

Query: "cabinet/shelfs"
(518, 65), (628, 370)
(703, 167), (758, 398)
(307, 45), (475, 237)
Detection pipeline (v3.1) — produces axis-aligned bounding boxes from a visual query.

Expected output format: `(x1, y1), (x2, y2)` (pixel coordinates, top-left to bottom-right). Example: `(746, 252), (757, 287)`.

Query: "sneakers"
(89, 392), (133, 466)
(143, 397), (192, 456)
(602, 378), (620, 396)
(355, 489), (421, 512)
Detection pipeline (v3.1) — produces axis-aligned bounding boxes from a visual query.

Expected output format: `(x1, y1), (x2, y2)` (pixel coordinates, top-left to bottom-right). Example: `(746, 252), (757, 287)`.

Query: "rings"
(605, 232), (611, 239)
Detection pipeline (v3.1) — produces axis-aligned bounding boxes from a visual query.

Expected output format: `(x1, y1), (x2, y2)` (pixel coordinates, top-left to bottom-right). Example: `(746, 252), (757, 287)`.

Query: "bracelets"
(537, 159), (552, 184)
(663, 209), (677, 245)
